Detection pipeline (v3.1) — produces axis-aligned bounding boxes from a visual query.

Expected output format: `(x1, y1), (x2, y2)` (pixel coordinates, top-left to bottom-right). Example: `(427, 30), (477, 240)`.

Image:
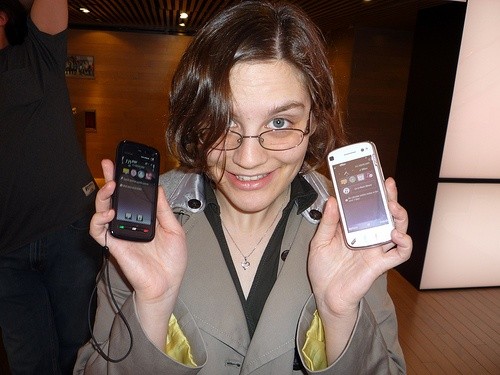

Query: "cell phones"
(109, 141), (160, 243)
(328, 141), (396, 250)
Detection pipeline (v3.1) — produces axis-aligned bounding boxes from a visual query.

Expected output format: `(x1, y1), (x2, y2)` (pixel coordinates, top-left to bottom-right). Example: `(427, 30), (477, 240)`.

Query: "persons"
(0, 0), (108, 375)
(74, 0), (416, 375)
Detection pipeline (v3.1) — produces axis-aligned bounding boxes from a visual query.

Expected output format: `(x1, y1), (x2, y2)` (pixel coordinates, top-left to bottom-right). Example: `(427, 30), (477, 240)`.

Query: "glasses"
(193, 113), (311, 151)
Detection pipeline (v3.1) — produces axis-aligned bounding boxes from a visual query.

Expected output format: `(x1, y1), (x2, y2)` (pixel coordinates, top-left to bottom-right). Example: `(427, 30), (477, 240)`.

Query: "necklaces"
(218, 206), (285, 270)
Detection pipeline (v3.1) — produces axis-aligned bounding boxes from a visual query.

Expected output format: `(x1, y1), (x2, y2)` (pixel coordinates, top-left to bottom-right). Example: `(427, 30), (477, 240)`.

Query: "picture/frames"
(64, 54), (95, 80)
